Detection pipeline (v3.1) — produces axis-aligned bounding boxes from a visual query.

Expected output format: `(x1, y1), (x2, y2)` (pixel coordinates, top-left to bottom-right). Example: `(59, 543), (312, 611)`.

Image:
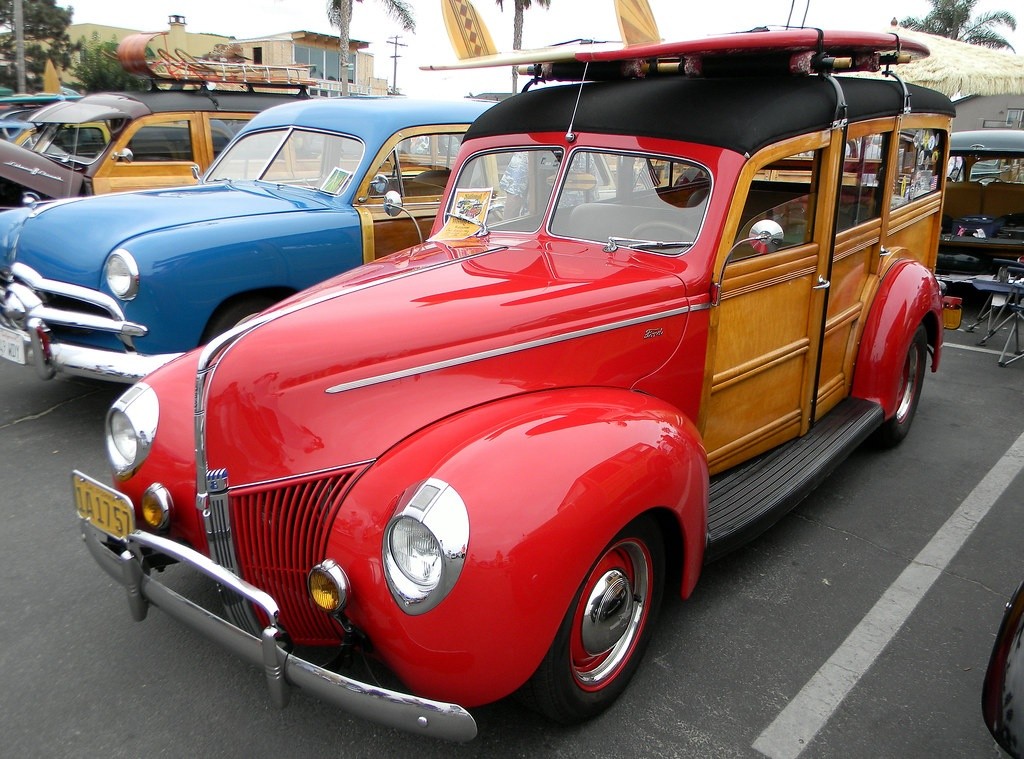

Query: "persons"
(749, 231), (771, 254)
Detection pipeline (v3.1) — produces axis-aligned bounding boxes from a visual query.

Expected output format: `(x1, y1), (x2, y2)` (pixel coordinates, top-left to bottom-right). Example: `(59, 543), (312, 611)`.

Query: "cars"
(0, 88), (464, 200)
(71, 21), (958, 743)
(1, 93), (615, 386)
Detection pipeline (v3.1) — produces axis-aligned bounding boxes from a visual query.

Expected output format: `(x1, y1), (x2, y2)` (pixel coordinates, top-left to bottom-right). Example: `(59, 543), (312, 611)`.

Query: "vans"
(932, 129), (1024, 306)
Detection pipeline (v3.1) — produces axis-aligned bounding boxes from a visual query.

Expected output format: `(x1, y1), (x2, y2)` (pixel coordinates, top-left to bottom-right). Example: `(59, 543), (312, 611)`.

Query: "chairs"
(967, 259), (1024, 366)
(409, 169), (451, 186)
(943, 182), (983, 216)
(984, 182), (1024, 218)
(569, 203), (680, 249)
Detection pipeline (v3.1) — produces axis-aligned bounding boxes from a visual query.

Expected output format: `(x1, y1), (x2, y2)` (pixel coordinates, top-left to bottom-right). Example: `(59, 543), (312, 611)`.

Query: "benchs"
(687, 189), (871, 242)
